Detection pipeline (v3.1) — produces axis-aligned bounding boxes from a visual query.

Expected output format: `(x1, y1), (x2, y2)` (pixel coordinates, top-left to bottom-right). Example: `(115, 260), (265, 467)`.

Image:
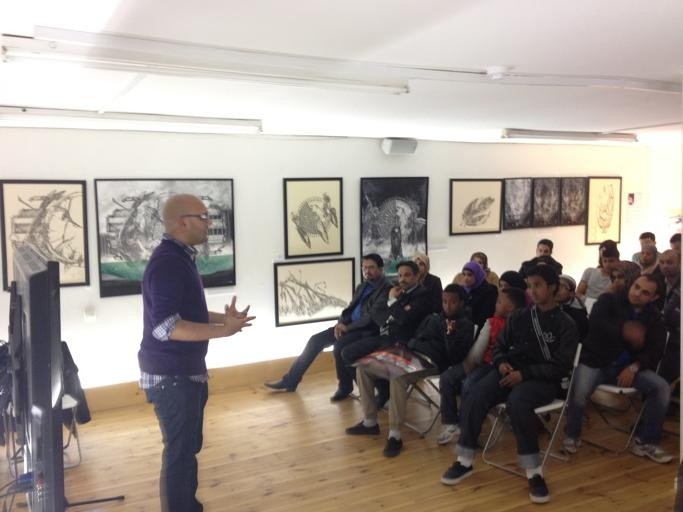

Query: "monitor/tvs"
(8, 243), (64, 512)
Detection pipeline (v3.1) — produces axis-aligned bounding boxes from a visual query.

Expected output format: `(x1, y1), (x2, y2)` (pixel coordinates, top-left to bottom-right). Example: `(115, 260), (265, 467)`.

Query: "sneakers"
(632, 438), (673, 463)
(346, 421), (380, 434)
(437, 425), (473, 485)
(383, 436), (403, 456)
(562, 436), (582, 454)
(528, 473), (549, 503)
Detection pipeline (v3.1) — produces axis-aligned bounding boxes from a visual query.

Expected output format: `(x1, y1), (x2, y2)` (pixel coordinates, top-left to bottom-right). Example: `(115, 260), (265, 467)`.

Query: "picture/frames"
(94, 178), (236, 298)
(503, 177), (589, 230)
(585, 176), (622, 246)
(449, 178), (504, 236)
(282, 177), (343, 259)
(274, 257), (356, 327)
(0, 179), (90, 292)
(359, 176), (429, 282)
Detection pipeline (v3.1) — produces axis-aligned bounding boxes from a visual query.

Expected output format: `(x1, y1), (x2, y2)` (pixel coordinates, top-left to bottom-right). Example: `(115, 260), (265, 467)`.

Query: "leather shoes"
(264, 378), (298, 391)
(331, 388), (353, 401)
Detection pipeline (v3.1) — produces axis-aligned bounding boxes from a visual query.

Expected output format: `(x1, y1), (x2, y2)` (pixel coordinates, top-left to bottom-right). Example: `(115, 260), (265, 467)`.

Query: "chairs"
(0, 342), (82, 476)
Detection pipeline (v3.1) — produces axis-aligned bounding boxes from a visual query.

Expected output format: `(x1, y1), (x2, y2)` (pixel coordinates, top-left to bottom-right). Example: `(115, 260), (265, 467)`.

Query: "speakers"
(382, 138), (418, 156)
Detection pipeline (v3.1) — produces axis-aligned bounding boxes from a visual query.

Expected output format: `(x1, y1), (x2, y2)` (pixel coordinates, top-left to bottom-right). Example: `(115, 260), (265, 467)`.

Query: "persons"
(343, 233), (683, 462)
(264, 253), (394, 400)
(138, 193), (256, 512)
(440, 265), (575, 504)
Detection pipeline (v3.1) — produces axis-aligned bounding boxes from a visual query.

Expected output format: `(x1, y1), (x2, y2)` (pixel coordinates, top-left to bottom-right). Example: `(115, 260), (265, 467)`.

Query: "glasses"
(181, 213), (208, 219)
(416, 262), (425, 266)
